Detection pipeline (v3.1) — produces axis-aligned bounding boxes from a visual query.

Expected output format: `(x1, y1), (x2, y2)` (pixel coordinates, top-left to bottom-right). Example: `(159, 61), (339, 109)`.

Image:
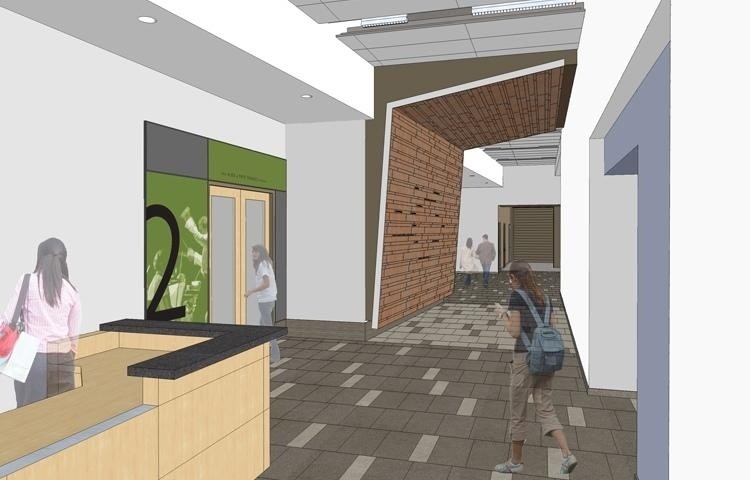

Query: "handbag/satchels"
(0, 330), (40, 383)
(0, 275), (31, 357)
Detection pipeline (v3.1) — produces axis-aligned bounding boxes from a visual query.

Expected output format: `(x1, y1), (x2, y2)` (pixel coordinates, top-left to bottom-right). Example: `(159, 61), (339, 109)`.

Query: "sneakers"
(494, 457), (523, 474)
(559, 453), (577, 475)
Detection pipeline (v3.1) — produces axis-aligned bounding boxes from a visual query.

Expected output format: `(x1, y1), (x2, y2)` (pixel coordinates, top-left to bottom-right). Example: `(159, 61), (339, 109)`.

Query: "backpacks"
(512, 288), (564, 376)
